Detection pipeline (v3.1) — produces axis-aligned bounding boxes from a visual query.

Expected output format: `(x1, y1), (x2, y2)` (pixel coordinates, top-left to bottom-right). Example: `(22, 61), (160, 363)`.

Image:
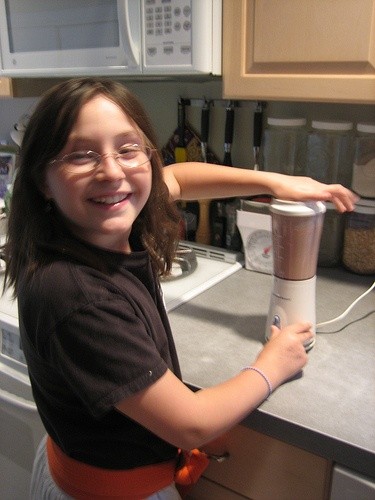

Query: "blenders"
(265, 197), (326, 351)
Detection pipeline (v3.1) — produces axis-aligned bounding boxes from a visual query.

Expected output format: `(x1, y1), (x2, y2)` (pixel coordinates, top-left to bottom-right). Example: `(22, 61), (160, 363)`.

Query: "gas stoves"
(0, 240), (245, 377)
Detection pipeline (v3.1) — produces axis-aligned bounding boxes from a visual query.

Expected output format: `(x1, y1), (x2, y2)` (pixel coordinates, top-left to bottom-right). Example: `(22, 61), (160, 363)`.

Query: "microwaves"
(0, 0), (222, 81)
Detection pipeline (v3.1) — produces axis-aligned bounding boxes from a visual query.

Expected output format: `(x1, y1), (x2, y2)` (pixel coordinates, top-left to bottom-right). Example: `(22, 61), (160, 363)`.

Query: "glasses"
(45, 143), (152, 174)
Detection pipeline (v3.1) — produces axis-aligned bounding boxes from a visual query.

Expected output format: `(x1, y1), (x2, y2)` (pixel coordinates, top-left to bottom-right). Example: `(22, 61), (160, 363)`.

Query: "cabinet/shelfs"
(178, 425), (333, 500)
(223, 0), (375, 104)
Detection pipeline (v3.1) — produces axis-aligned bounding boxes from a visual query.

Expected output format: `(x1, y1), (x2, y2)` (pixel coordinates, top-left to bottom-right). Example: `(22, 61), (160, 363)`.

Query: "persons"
(0, 78), (361, 500)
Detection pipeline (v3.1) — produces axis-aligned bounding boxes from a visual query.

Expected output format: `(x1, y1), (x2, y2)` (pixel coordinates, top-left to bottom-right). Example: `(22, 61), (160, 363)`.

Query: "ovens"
(0, 361), (47, 500)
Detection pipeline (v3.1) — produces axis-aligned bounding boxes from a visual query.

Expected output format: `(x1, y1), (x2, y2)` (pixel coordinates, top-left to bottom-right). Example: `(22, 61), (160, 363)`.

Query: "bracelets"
(243, 367), (272, 395)
